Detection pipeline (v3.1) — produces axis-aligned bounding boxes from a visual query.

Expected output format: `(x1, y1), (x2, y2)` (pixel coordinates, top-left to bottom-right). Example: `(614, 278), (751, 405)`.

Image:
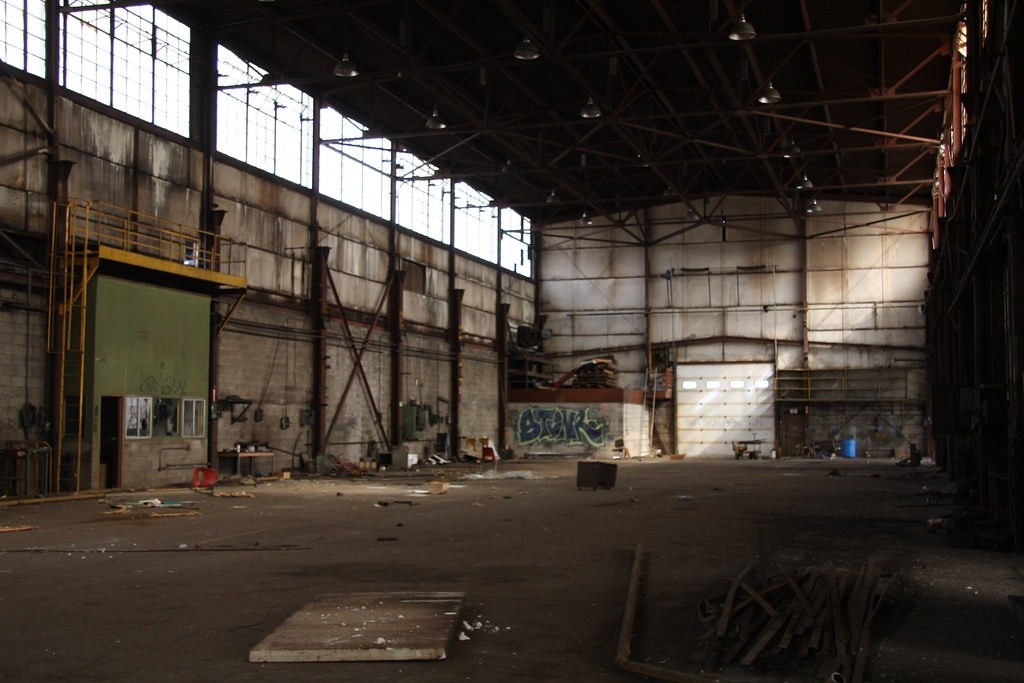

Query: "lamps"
(757, 81), (782, 105)
(546, 190), (562, 202)
(580, 95), (602, 118)
(795, 173), (814, 189)
(577, 211), (593, 224)
(333, 52), (360, 77)
(685, 208), (699, 221)
(783, 136), (802, 159)
(728, 0), (756, 41)
(662, 185), (677, 197)
(501, 159), (516, 172)
(424, 110), (447, 129)
(513, 36), (540, 59)
(805, 198), (822, 213)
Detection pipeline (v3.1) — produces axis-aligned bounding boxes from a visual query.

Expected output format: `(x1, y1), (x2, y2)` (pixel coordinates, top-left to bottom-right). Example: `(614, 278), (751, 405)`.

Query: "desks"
(217, 451), (275, 476)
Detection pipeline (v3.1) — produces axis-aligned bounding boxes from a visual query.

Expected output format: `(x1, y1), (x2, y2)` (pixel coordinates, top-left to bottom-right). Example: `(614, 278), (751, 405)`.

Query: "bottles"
(237, 442), (241, 453)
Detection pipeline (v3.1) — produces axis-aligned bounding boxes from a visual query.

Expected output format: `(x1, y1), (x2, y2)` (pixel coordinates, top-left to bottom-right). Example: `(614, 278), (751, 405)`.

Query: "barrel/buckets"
(841, 437), (856, 457)
